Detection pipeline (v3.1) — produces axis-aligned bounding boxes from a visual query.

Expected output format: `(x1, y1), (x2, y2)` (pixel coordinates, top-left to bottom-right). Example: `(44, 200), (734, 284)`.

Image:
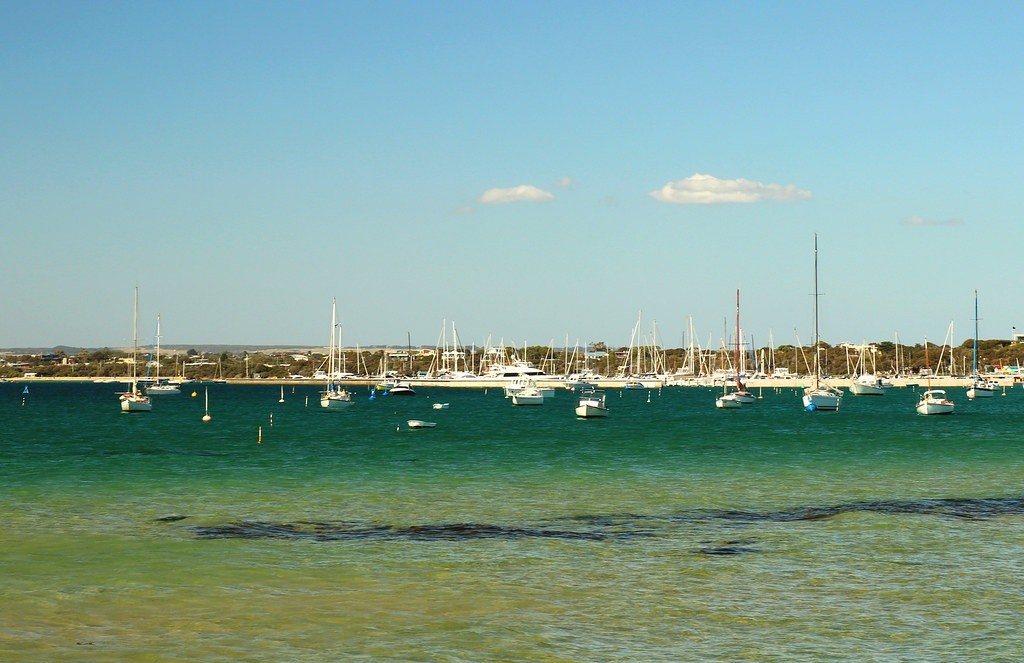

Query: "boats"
(407, 419), (437, 429)
(575, 394), (611, 417)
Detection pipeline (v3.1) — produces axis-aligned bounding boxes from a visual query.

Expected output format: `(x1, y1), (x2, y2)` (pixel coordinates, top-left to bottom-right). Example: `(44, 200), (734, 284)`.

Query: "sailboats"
(283, 296), (972, 409)
(279, 385), (285, 403)
(119, 286), (152, 411)
(202, 386), (212, 422)
(143, 313), (182, 395)
(966, 290), (994, 399)
(915, 334), (955, 415)
(729, 288), (757, 404)
(167, 352), (193, 384)
(802, 232), (844, 410)
(715, 337), (742, 408)
(212, 358), (226, 384)
